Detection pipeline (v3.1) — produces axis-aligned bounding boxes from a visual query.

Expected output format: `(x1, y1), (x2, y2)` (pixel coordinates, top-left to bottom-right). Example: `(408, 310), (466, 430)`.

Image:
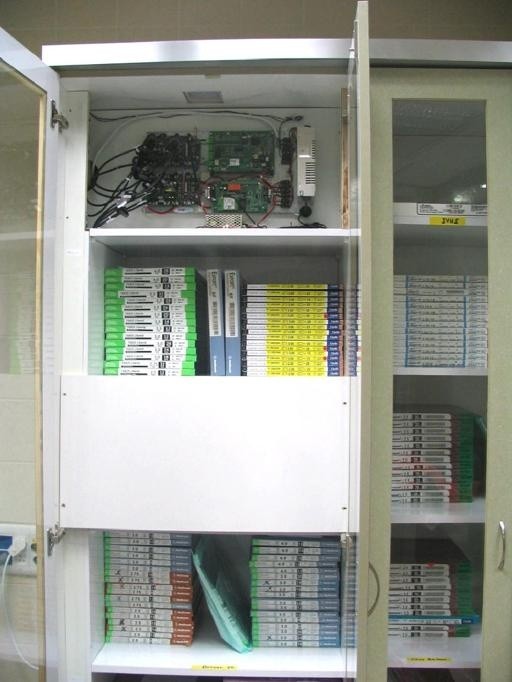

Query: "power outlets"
(0, 523), (36, 579)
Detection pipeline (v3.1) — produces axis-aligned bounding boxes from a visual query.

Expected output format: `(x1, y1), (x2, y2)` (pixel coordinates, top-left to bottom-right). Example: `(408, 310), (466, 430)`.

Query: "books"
(224, 271), (243, 377)
(246, 283), (344, 376)
(103, 530), (200, 646)
(394, 203), (488, 217)
(391, 403), (474, 503)
(474, 415), (488, 498)
(388, 537), (480, 637)
(206, 268), (225, 376)
(393, 274), (488, 368)
(104, 266), (197, 376)
(248, 535), (341, 648)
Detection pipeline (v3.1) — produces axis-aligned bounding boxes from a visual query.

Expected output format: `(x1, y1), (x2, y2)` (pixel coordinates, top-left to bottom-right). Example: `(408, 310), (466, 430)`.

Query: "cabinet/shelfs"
(2, 0), (373, 682)
(368, 35), (511, 682)
